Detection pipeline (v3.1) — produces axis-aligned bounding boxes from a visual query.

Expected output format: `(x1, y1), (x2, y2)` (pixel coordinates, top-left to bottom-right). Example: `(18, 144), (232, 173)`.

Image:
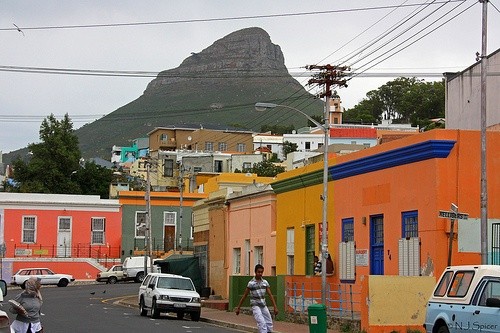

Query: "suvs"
(138, 273), (202, 322)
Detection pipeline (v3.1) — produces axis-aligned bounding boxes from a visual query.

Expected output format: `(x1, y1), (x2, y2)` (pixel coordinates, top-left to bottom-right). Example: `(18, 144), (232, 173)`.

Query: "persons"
(21, 280), (45, 316)
(8, 277), (43, 333)
(327, 254), (334, 274)
(139, 218), (145, 225)
(314, 256), (322, 275)
(164, 232), (183, 255)
(236, 264), (279, 333)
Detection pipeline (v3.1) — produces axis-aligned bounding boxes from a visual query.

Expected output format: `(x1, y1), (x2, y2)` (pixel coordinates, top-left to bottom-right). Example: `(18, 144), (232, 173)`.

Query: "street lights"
(254, 102), (330, 305)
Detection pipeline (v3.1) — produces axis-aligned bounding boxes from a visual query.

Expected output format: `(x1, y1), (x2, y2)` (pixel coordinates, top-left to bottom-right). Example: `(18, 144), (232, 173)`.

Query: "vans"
(122, 256), (164, 283)
(423, 265), (500, 333)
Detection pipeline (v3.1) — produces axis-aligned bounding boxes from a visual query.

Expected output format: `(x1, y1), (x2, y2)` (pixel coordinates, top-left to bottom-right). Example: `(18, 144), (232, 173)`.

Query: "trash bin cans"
(307, 303), (328, 333)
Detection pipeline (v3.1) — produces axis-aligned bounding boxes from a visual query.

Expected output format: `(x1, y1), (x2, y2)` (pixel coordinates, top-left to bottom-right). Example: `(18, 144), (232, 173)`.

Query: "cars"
(10, 268), (75, 287)
(95, 265), (133, 284)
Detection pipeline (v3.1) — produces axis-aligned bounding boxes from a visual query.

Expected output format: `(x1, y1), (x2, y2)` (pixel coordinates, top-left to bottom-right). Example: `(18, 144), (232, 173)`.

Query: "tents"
(155, 253), (205, 298)
